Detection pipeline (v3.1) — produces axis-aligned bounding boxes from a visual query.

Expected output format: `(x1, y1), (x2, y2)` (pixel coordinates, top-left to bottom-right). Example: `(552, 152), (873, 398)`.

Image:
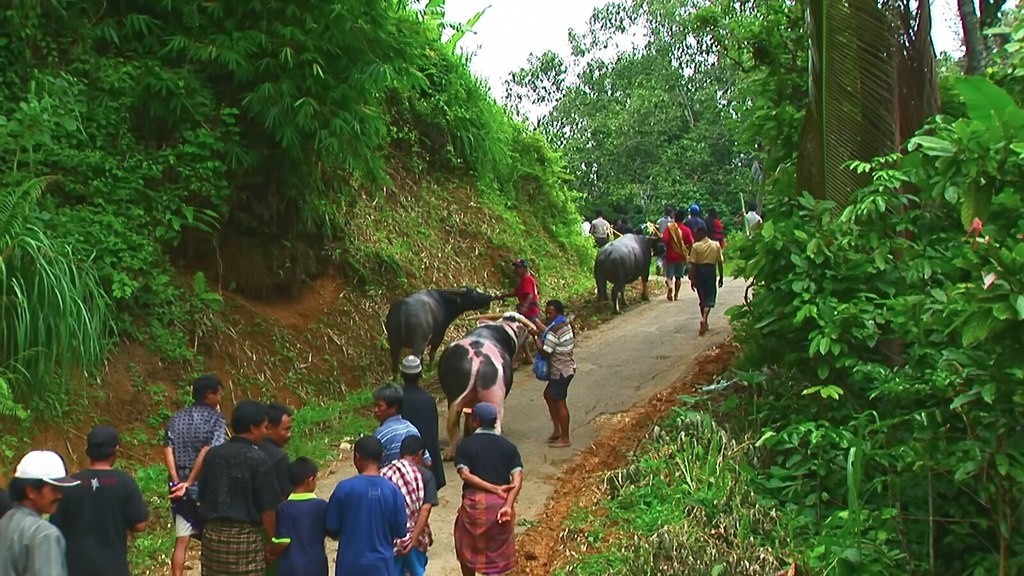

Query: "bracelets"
(168, 481), (180, 487)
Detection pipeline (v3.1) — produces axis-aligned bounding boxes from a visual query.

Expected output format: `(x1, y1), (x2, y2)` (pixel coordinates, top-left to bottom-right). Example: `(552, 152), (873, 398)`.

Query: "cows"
(435, 310), (539, 464)
(384, 282), (495, 385)
(592, 231), (663, 316)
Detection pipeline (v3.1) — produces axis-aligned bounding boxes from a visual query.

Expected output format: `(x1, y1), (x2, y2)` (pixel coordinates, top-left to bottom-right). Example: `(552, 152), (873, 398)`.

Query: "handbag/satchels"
(534, 356), (549, 380)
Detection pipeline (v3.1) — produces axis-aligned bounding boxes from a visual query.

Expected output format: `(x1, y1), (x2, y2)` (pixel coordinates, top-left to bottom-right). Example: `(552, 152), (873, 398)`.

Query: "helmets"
(690, 205), (699, 213)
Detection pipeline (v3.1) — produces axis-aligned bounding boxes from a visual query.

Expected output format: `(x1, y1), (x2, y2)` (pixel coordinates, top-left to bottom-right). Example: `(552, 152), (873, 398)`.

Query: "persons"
(497, 260), (548, 364)
(163, 375), (227, 576)
(325, 435), (408, 576)
(734, 201), (763, 230)
(0, 450), (82, 576)
(578, 201), (724, 302)
(530, 299), (577, 448)
(453, 401), (523, 576)
(197, 399), (293, 576)
(371, 355), (446, 515)
(689, 227), (724, 335)
(378, 434), (434, 576)
(47, 425), (149, 576)
(270, 457), (328, 576)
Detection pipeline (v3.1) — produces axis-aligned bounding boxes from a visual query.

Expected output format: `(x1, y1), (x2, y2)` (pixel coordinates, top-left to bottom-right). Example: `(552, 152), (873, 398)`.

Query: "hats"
(463, 402), (497, 421)
(14, 450), (81, 486)
(398, 355), (422, 373)
(87, 427), (118, 449)
(512, 259), (527, 267)
